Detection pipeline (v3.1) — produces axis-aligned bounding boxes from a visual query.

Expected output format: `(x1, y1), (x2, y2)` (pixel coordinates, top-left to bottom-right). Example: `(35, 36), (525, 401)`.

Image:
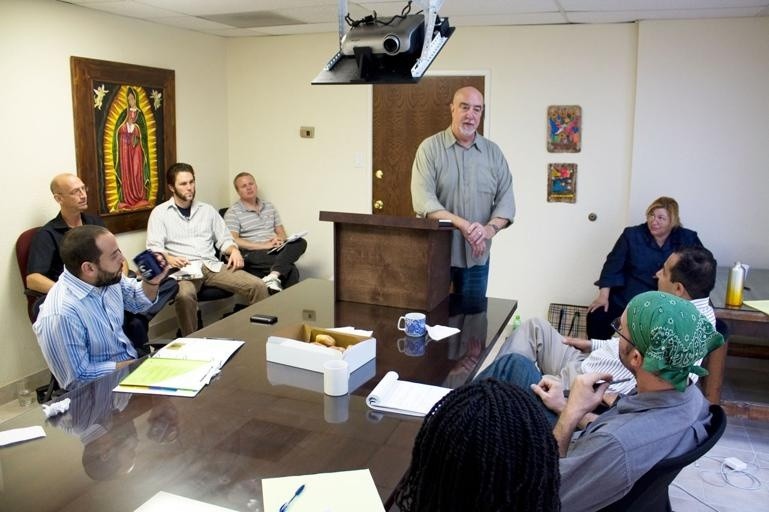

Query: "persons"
(471, 291), (725, 512)
(23, 172), (180, 358)
(115, 83), (152, 209)
(47, 353), (181, 482)
(409, 85), (516, 303)
(396, 377), (562, 512)
(493, 245), (718, 395)
(442, 296), (488, 390)
(31, 224), (171, 394)
(145, 162), (271, 339)
(223, 171), (308, 296)
(585, 196), (703, 340)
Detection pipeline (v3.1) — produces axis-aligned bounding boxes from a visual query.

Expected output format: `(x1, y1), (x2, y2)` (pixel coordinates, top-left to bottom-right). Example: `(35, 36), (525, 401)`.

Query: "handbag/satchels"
(548, 303), (589, 340)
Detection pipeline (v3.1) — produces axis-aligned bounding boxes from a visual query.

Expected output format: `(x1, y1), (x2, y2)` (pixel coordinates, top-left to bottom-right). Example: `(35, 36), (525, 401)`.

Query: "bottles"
(513, 315), (522, 330)
(726, 261), (745, 306)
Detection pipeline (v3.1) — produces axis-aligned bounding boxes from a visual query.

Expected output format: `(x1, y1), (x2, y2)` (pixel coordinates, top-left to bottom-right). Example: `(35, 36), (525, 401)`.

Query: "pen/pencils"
(279, 482), (307, 512)
(593, 378), (633, 388)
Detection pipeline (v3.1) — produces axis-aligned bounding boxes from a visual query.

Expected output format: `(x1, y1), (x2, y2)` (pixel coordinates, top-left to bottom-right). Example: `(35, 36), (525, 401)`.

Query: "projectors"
(339, 12), (426, 60)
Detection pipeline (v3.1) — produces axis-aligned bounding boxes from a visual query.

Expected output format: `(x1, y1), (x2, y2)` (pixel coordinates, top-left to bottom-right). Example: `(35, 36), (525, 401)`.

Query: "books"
(364, 370), (455, 419)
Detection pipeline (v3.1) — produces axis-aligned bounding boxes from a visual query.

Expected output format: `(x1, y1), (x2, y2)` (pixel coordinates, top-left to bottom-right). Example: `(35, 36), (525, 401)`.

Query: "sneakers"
(261, 275), (282, 295)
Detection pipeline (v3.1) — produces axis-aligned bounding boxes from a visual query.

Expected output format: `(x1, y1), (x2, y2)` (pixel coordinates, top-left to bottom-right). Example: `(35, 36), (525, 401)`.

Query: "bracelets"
(489, 221), (501, 235)
(143, 279), (158, 287)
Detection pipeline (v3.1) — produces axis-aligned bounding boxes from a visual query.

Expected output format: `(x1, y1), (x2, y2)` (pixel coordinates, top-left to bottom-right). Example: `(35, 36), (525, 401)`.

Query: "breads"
(308, 333), (354, 353)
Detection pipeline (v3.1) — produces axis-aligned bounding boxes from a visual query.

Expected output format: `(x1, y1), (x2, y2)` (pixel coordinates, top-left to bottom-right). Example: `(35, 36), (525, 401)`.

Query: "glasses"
(610, 317), (645, 358)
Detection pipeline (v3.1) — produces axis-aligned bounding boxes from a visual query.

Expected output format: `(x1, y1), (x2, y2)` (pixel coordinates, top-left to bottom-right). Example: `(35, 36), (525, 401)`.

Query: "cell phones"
(249, 313), (278, 324)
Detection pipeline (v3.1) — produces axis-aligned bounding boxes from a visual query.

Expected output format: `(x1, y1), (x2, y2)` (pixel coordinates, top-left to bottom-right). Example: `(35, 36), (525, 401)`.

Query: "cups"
(397, 338), (425, 357)
(397, 312), (427, 337)
(145, 417), (180, 444)
(323, 360), (351, 396)
(133, 248), (167, 282)
(324, 395), (349, 424)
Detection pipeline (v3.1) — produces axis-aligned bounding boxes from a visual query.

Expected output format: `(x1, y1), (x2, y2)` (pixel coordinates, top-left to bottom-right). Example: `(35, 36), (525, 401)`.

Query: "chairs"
(16, 226), (69, 405)
(169, 206), (231, 337)
(602, 403), (726, 511)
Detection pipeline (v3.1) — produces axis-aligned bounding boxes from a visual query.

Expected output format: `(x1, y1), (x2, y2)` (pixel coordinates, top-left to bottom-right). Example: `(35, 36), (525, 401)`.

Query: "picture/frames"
(69, 54), (177, 233)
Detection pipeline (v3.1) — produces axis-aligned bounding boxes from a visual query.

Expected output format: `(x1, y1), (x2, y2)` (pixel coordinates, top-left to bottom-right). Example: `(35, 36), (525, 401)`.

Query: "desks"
(700, 267), (769, 421)
(1, 277), (517, 511)
